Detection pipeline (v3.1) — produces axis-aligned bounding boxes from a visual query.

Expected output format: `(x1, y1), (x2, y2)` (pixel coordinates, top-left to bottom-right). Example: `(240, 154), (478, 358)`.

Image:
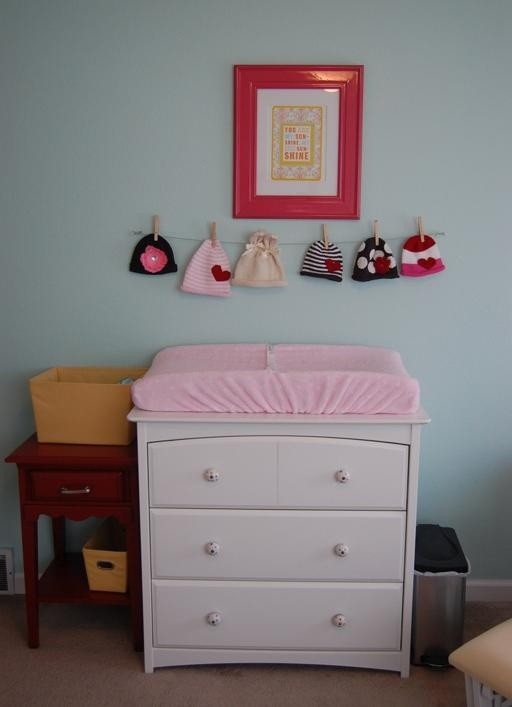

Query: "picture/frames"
(233, 64), (364, 219)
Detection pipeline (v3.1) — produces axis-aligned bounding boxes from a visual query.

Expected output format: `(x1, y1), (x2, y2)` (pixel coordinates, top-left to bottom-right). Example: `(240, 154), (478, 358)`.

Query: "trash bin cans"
(414, 523), (473, 672)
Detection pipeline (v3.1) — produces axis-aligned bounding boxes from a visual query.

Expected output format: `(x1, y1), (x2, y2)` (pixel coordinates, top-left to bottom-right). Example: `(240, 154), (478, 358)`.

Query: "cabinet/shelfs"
(5, 432), (143, 652)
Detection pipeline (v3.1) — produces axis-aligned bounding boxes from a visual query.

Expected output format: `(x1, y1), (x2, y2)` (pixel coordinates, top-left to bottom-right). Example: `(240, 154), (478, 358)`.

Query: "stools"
(126, 405), (432, 679)
(448, 619), (512, 707)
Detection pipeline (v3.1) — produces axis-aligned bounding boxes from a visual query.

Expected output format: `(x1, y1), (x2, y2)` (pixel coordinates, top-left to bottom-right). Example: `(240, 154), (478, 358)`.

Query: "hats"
(129, 234), (177, 275)
(401, 235), (445, 276)
(300, 240), (343, 282)
(352, 238), (400, 280)
(231, 233), (287, 286)
(180, 239), (229, 298)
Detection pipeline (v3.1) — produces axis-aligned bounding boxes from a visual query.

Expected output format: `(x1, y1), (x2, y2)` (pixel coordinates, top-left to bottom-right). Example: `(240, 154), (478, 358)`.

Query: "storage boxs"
(82, 518), (127, 594)
(28, 367), (148, 446)
